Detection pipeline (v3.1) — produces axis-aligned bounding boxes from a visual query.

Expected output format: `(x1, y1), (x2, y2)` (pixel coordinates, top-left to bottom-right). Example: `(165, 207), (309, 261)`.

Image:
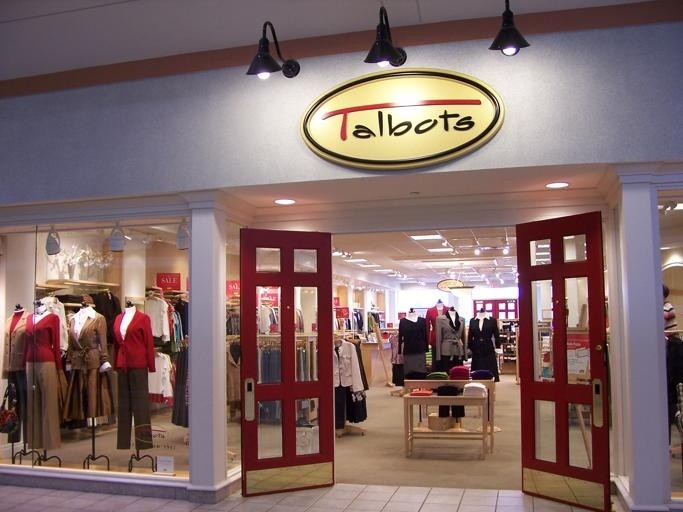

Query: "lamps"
(441, 243), (514, 260)
(241, 16), (303, 84)
(356, 6), (413, 71)
(38, 220), (193, 258)
(446, 267), (475, 298)
(485, 1), (532, 62)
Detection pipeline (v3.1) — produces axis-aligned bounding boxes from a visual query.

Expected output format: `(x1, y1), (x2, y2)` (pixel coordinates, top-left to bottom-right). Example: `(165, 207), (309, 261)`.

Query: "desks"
(404, 376), (494, 454)
(402, 391), (487, 460)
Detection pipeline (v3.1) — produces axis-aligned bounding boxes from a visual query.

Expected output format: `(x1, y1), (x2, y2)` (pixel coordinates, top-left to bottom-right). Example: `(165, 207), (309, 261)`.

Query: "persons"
(397, 299), (503, 418)
(3, 301), (156, 450)
(662, 285), (680, 337)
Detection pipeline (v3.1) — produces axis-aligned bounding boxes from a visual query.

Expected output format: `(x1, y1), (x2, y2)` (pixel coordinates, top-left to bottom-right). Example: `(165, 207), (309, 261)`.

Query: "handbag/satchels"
(0, 382), (18, 433)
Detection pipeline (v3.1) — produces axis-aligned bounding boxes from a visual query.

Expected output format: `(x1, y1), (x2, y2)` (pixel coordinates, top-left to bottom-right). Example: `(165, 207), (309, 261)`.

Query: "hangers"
(143, 284), (360, 356)
